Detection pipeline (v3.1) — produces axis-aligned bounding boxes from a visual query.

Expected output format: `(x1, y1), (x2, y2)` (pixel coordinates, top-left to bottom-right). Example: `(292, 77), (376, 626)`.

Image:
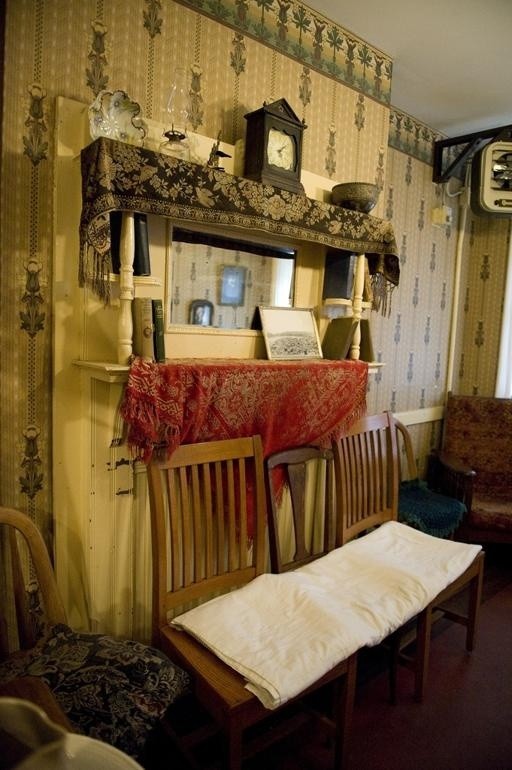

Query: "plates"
(88, 88), (146, 147)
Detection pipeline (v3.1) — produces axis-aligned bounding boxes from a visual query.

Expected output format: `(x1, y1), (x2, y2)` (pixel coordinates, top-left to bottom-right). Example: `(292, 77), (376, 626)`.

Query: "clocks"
(243, 98), (308, 195)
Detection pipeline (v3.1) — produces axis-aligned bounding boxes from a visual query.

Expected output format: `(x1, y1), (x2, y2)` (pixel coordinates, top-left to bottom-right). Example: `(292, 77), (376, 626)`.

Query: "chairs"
(144, 435), (361, 769)
(392, 422), (468, 542)
(328, 410), (485, 705)
(429, 391), (511, 543)
(0, 506), (199, 761)
(263, 446), (424, 742)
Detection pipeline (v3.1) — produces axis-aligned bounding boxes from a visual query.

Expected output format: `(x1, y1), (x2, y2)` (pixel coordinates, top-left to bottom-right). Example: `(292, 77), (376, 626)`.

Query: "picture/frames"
(218, 264), (248, 308)
(258, 305), (324, 361)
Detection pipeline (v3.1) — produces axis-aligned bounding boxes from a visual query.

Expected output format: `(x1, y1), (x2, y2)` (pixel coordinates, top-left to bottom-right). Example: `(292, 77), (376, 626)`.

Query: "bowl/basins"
(331, 183), (382, 213)
